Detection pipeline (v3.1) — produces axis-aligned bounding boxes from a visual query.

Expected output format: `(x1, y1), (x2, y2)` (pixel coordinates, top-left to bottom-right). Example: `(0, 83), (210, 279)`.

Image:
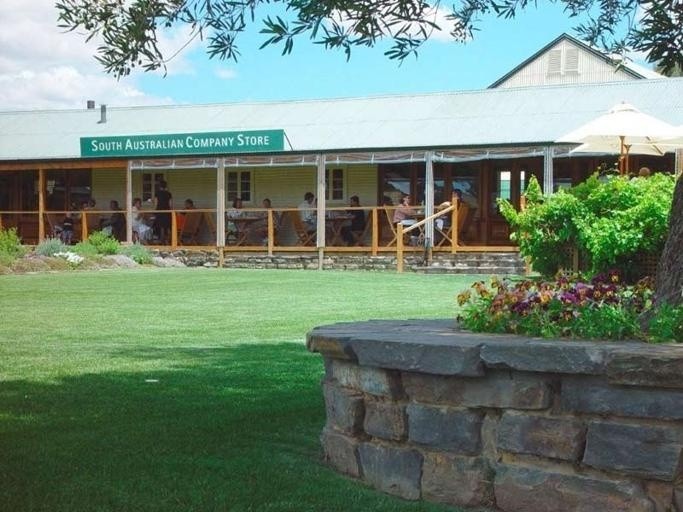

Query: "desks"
(407, 213), (447, 248)
(319, 214), (353, 246)
(227, 215), (263, 248)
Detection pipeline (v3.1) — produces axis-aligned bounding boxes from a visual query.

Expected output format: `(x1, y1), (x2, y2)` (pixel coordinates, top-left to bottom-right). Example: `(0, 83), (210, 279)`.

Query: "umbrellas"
(566, 142), (676, 178)
(553, 98), (682, 179)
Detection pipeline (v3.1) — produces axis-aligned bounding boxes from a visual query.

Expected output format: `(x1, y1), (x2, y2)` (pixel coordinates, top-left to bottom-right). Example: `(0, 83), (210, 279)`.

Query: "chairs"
(350, 205), (410, 247)
(441, 204), (469, 249)
(45, 209), (127, 245)
(175, 207), (231, 248)
(263, 206), (317, 248)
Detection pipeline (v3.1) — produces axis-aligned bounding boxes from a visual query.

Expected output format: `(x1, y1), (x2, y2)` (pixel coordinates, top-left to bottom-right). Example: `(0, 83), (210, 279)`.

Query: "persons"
(391, 194), (420, 246)
(53, 180), (198, 246)
(433, 188), (465, 245)
(254, 198), (281, 247)
(224, 198), (251, 246)
(296, 191), (317, 245)
(340, 195), (366, 246)
